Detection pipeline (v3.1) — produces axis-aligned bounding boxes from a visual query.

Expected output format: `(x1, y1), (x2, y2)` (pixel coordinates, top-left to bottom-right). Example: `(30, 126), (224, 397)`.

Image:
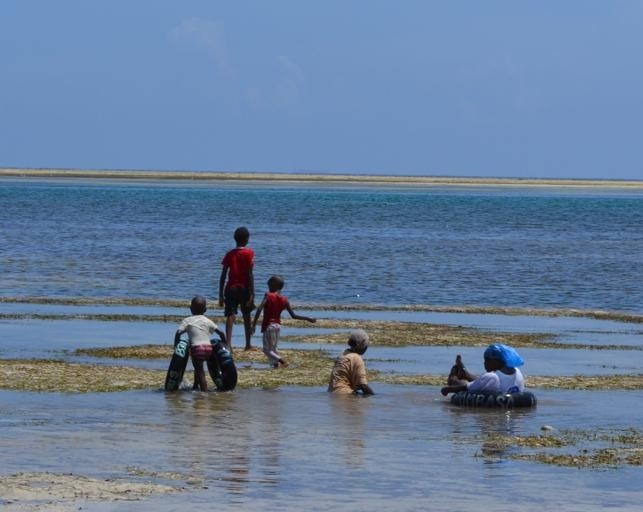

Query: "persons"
(327, 328), (376, 395)
(217, 226), (257, 353)
(173, 296), (228, 391)
(441, 342), (525, 397)
(245, 275), (316, 369)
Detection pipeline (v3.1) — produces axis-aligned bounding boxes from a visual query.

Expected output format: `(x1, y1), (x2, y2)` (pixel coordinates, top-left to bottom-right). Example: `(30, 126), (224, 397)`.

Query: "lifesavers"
(164, 338), (188, 390)
(206, 338), (237, 390)
(451, 390), (536, 409)
